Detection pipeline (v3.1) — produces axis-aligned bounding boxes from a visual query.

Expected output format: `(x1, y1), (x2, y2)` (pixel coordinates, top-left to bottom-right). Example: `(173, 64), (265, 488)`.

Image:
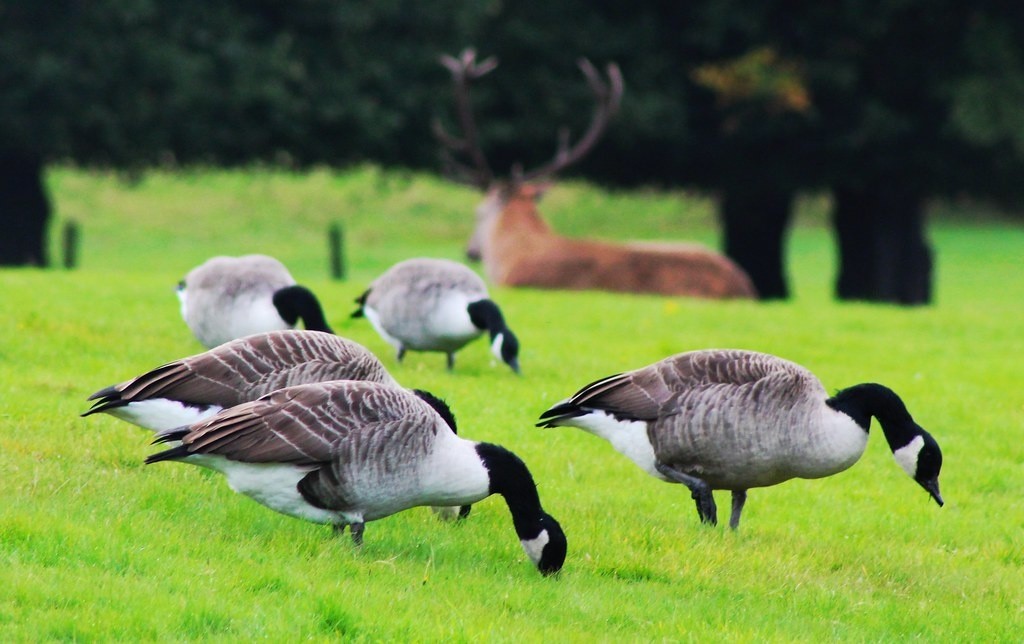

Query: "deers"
(432, 49), (763, 301)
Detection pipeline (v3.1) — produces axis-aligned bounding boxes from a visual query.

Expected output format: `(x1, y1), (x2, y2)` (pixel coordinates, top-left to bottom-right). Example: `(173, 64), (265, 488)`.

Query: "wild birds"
(532, 348), (944, 529)
(350, 256), (523, 372)
(176, 250), (337, 348)
(138, 378), (568, 579)
(77, 329), (474, 523)
